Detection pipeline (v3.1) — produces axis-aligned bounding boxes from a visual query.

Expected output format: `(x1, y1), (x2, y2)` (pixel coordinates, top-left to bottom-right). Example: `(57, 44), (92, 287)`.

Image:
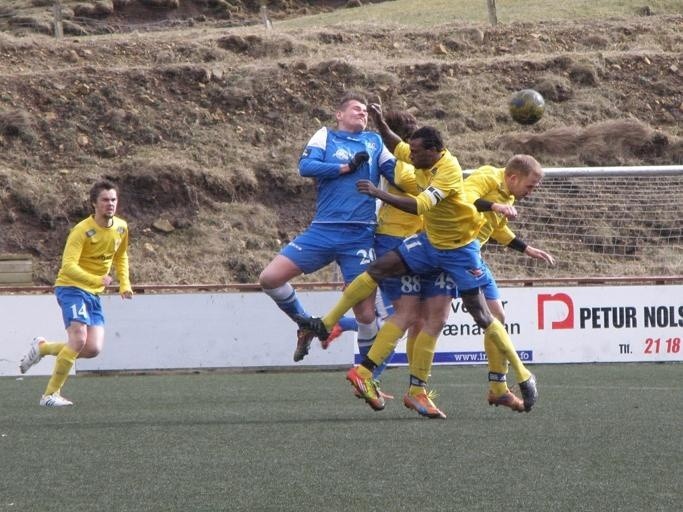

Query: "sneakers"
(19, 336), (46, 373)
(40, 394), (72, 407)
(293, 315), (538, 418)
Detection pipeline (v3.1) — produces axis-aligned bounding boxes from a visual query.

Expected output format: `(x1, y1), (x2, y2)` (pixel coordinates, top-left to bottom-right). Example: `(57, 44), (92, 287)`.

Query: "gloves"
(349, 151), (369, 173)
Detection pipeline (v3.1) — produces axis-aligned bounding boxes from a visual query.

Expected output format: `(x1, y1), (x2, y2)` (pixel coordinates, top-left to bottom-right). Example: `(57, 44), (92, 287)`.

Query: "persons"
(20, 180), (134, 408)
(257, 91), (556, 420)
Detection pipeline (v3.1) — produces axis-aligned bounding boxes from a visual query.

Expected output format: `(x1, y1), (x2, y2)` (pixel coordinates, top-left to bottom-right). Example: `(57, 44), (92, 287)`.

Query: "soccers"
(510, 89), (545, 124)
